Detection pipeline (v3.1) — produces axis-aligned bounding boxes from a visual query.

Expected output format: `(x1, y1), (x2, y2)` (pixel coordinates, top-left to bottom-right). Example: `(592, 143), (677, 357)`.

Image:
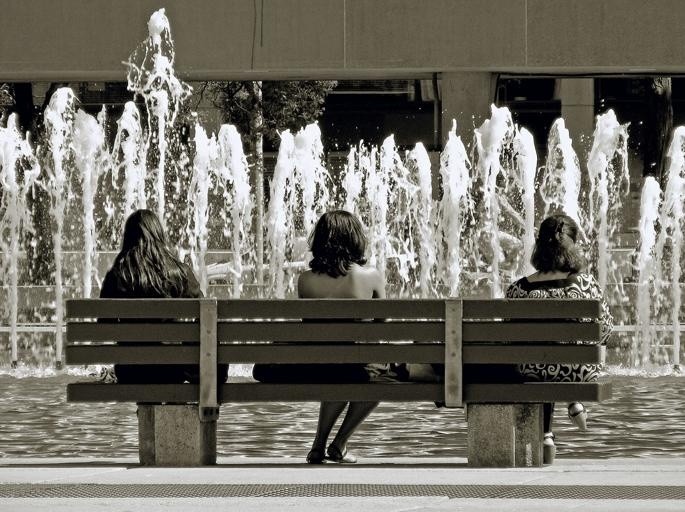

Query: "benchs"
(62, 297), (604, 470)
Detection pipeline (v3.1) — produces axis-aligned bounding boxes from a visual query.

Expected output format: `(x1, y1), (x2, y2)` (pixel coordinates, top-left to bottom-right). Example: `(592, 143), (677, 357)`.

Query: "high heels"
(568, 402), (588, 430)
(543, 434), (556, 464)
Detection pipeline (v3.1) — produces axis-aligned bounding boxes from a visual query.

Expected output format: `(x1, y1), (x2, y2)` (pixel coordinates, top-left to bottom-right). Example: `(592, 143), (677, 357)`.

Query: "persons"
(501, 214), (615, 464)
(295, 208), (394, 465)
(467, 176), (526, 268)
(94, 208), (230, 419)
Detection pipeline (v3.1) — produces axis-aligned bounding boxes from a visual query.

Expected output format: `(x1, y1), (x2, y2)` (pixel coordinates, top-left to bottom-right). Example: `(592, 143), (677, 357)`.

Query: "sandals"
(325, 443), (357, 463)
(306, 447), (327, 464)
